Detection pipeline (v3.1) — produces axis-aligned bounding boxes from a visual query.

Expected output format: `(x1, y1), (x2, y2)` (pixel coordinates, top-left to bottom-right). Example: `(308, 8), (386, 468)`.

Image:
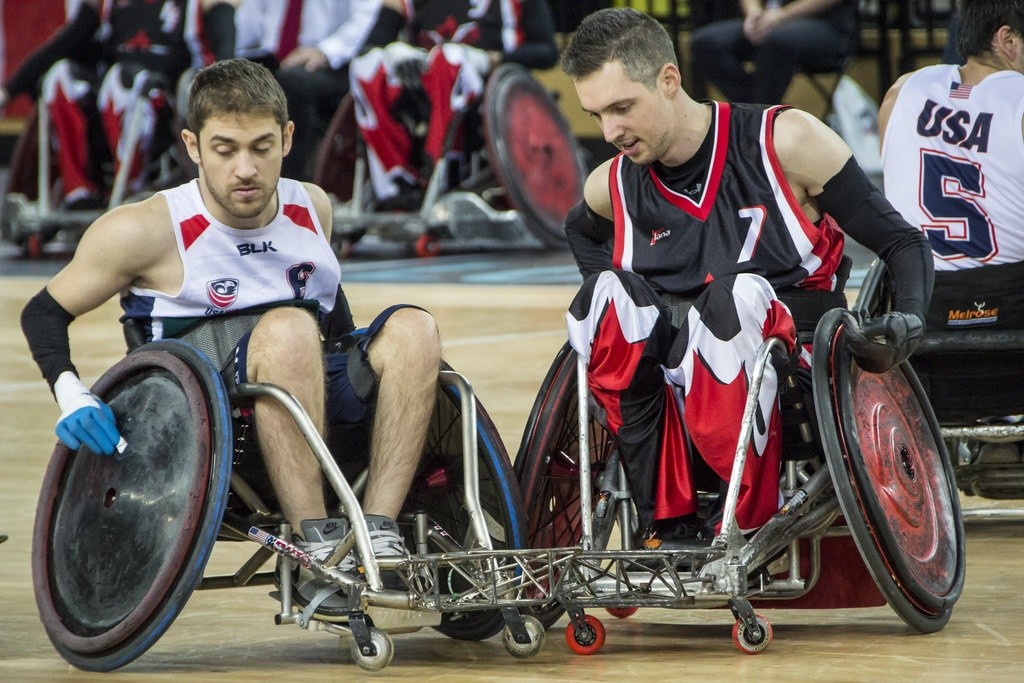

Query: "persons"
(561, 8), (936, 581)
(692, 0), (861, 105)
(878, 0), (1024, 270)
(19, 58), (441, 616)
(0, 0), (560, 255)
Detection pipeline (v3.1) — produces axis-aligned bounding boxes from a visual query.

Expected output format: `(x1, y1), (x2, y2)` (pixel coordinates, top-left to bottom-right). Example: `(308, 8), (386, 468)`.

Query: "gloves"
(385, 40), (424, 88)
(843, 310), (923, 374)
(54, 377), (120, 456)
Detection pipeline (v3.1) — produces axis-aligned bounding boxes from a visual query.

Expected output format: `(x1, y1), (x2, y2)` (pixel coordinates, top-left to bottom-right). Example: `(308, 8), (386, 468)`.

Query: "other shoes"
(635, 518), (714, 569)
(377, 187), (425, 211)
(63, 189), (91, 209)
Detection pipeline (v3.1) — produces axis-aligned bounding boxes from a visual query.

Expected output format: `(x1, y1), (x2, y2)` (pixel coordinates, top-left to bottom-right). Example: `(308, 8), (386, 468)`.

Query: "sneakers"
(354, 514), (411, 591)
(274, 518), (361, 622)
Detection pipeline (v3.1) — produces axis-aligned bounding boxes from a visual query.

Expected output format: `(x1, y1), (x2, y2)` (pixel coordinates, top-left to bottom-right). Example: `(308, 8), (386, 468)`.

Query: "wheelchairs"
(515, 256), (969, 651)
(314, 60), (587, 255)
(0, 48), (171, 261)
(849, 255), (1023, 527)
(29, 306), (520, 675)
(173, 65), (373, 258)
